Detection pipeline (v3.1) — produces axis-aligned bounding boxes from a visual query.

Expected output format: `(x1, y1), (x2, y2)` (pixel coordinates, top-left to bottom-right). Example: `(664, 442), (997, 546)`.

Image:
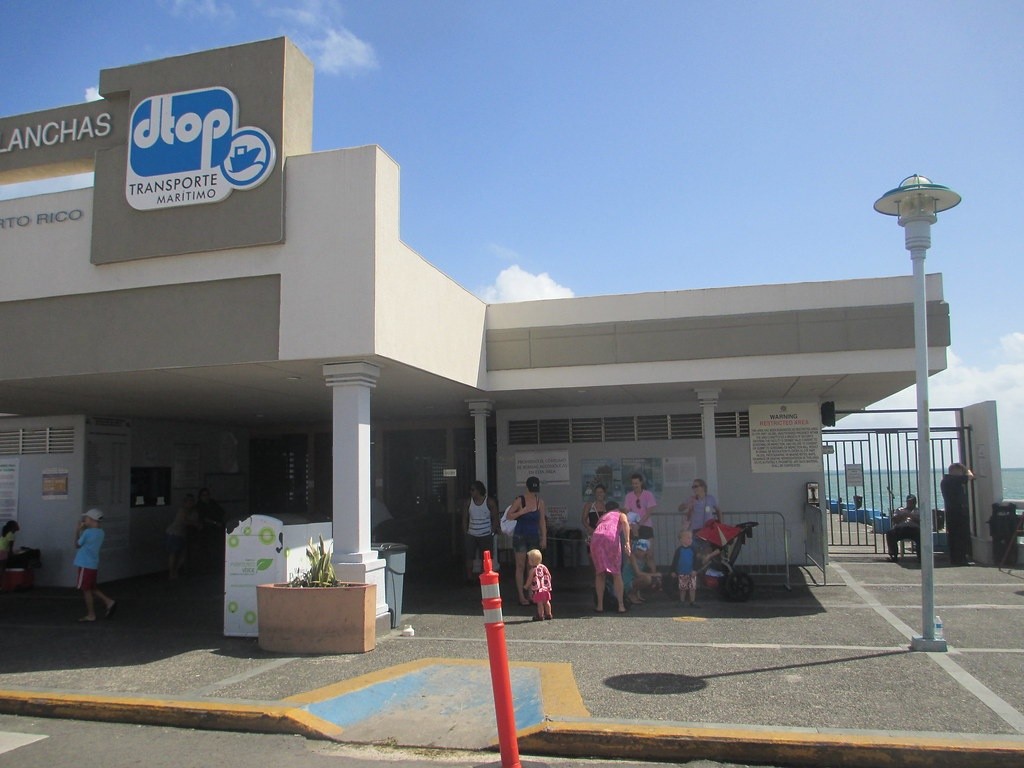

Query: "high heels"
(626, 590), (646, 603)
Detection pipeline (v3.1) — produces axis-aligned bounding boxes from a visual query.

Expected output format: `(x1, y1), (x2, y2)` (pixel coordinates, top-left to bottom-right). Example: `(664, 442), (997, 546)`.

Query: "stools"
(901, 538), (917, 561)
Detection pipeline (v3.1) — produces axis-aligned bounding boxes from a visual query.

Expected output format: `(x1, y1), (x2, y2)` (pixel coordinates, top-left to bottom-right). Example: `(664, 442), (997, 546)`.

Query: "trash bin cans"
(371, 542), (408, 627)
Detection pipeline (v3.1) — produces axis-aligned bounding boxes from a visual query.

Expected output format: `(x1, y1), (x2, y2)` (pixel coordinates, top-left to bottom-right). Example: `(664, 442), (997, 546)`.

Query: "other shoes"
(533, 615), (553, 621)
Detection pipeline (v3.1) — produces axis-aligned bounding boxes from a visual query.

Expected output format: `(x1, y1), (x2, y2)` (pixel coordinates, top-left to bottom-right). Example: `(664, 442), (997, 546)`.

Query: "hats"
(906, 495), (916, 503)
(526, 477), (541, 493)
(636, 538), (651, 552)
(82, 508), (105, 522)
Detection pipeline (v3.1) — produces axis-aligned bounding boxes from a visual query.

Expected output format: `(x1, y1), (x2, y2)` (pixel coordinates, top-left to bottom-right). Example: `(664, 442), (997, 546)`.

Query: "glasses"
(692, 484), (702, 488)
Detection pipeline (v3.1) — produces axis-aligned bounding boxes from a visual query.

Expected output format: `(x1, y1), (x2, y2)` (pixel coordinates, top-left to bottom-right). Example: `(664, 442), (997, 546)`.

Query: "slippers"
(77, 615), (98, 622)
(106, 600), (118, 618)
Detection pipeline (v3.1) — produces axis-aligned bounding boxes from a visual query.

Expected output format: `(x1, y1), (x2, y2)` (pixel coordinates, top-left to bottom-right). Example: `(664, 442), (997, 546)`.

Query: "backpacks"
(500, 495), (526, 536)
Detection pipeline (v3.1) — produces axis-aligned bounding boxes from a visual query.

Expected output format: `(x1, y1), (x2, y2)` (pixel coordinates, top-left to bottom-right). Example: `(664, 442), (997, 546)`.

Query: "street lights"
(872, 173), (962, 641)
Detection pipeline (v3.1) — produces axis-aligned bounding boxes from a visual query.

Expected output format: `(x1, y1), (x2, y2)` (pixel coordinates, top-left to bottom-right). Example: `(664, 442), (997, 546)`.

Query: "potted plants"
(254, 532), (378, 655)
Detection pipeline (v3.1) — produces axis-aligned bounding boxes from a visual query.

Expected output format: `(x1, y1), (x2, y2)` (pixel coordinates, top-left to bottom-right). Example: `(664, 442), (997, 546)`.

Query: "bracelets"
(214, 521), (216, 525)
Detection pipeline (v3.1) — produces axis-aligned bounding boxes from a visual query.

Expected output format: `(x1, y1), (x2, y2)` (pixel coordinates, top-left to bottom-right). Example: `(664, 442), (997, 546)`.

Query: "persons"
(0, 521), (20, 592)
(884, 495), (923, 564)
(461, 481), (497, 588)
(506, 476), (548, 606)
(163, 489), (227, 588)
(524, 549), (554, 621)
(73, 507), (117, 623)
(941, 464), (975, 566)
(581, 474), (723, 614)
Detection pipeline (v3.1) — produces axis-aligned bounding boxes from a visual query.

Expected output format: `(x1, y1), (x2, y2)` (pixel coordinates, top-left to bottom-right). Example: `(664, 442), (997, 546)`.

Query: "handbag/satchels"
(585, 530), (594, 544)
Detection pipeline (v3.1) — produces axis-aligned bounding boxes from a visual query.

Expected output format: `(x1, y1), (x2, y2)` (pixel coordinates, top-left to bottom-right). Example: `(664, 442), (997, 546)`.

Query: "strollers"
(675, 519), (760, 597)
